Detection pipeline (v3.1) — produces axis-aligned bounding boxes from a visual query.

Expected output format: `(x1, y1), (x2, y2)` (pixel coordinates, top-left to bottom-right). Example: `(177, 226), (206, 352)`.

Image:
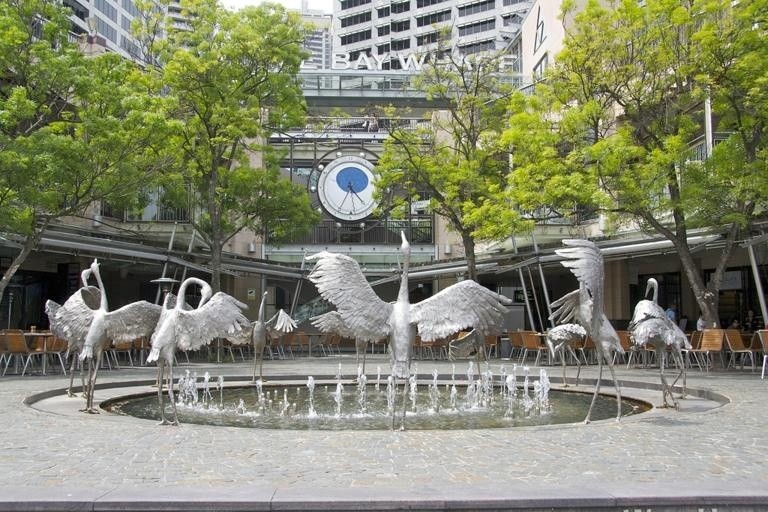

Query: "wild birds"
(226, 287), (298, 386)
(539, 237), (695, 427)
(43, 256), (253, 427)
(304, 229), (511, 428)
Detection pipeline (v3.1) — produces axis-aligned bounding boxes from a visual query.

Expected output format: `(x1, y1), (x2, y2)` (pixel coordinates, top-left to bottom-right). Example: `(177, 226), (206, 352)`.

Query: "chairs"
(0, 319), (767, 378)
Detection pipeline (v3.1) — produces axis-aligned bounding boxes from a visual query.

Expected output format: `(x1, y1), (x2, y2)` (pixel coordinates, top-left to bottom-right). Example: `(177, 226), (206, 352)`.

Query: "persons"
(665, 302), (759, 332)
(362, 113), (379, 132)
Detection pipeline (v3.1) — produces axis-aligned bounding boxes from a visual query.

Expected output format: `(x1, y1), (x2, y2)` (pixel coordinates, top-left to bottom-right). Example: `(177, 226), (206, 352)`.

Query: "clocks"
(306, 145), (393, 236)
